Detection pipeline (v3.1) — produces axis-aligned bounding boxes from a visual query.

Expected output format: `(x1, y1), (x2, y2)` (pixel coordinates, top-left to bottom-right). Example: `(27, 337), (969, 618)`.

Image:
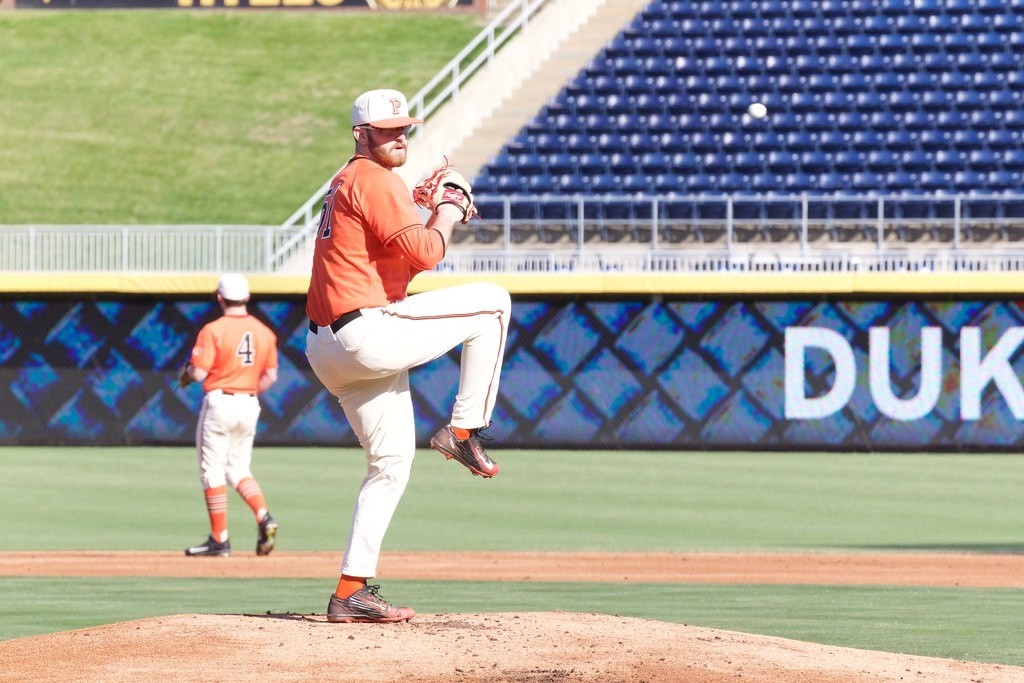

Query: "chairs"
(450, 0), (1024, 242)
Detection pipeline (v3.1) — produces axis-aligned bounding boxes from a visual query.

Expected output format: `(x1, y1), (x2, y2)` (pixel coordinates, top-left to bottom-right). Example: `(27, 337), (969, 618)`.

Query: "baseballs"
(749, 102), (767, 119)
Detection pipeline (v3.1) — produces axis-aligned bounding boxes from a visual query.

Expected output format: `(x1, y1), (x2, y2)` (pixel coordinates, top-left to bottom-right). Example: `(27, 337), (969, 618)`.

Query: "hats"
(218, 275), (250, 301)
(351, 89), (424, 129)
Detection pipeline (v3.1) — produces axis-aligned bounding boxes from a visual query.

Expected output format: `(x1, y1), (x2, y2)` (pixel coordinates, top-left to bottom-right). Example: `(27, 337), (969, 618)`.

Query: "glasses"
(356, 125), (412, 137)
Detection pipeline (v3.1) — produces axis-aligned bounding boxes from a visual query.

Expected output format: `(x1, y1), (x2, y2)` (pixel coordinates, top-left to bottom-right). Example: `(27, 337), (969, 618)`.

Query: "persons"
(179, 273), (277, 557)
(304, 89), (512, 623)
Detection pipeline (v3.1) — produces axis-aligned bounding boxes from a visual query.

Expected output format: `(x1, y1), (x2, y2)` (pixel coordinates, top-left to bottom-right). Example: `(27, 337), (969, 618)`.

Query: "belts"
(223, 390), (255, 397)
(309, 309), (362, 336)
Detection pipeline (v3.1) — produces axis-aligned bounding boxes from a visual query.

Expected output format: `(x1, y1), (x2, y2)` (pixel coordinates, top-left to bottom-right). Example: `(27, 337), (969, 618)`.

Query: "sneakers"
(328, 581), (415, 623)
(430, 421), (499, 479)
(256, 513), (277, 555)
(185, 534), (231, 558)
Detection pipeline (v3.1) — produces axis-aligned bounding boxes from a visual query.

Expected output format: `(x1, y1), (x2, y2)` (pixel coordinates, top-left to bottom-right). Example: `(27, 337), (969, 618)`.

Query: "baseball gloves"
(178, 360), (192, 388)
(412, 168), (476, 224)
